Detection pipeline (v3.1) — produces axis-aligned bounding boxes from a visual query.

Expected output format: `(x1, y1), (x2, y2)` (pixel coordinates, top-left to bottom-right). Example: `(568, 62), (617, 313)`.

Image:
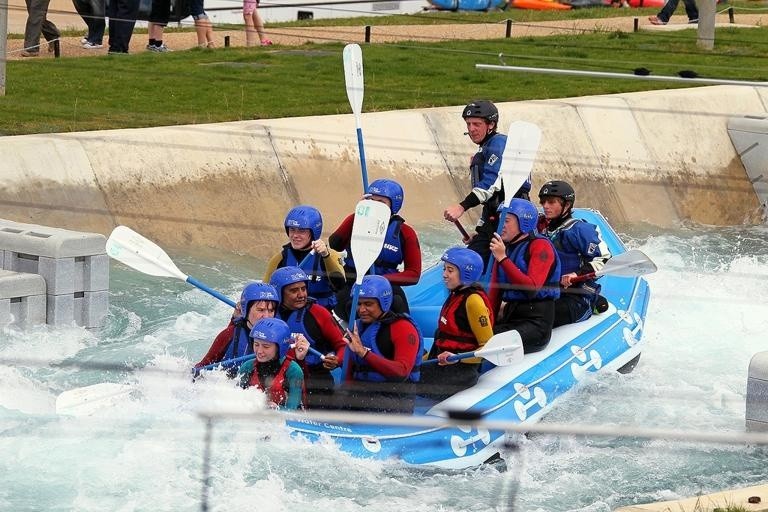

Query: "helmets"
(241, 283), (279, 319)
(538, 181), (575, 208)
(269, 266), (310, 307)
(248, 317), (291, 357)
(349, 275), (393, 312)
(440, 247), (484, 286)
(462, 101), (498, 124)
(369, 179), (404, 215)
(496, 198), (539, 234)
(284, 206), (322, 240)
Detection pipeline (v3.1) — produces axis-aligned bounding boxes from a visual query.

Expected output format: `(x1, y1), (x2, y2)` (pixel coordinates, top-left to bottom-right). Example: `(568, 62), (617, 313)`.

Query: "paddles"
(561, 250), (658, 285)
(105, 226), (335, 360)
(55, 342), (299, 416)
(338, 200), (392, 385)
(420, 330), (525, 367)
(483, 121), (540, 293)
(343, 44), (374, 275)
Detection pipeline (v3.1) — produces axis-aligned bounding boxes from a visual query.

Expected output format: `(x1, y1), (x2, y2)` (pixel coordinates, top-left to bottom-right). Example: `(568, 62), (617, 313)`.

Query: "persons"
(237, 318), (305, 416)
(416, 247), (494, 400)
(647, 0), (699, 25)
(262, 205), (346, 311)
(18, 0), (280, 58)
(538, 179), (612, 328)
(443, 101), (532, 269)
(329, 179), (421, 313)
(342, 274), (424, 383)
(229, 266), (347, 383)
(489, 198), (562, 353)
(195, 282), (311, 378)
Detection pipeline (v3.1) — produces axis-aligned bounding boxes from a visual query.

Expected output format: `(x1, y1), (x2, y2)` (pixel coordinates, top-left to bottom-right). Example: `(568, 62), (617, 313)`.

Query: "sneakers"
(22, 51), (39, 56)
(649, 17), (667, 25)
(82, 36), (89, 43)
(83, 42), (103, 48)
(149, 43), (173, 51)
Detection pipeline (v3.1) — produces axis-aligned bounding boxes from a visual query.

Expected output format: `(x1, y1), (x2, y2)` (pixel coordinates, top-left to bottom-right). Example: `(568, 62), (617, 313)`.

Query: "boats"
(228, 206), (648, 472)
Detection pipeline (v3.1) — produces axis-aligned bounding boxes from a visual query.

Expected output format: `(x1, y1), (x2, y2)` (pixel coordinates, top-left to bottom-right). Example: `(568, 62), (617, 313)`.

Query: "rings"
(332, 357), (335, 359)
